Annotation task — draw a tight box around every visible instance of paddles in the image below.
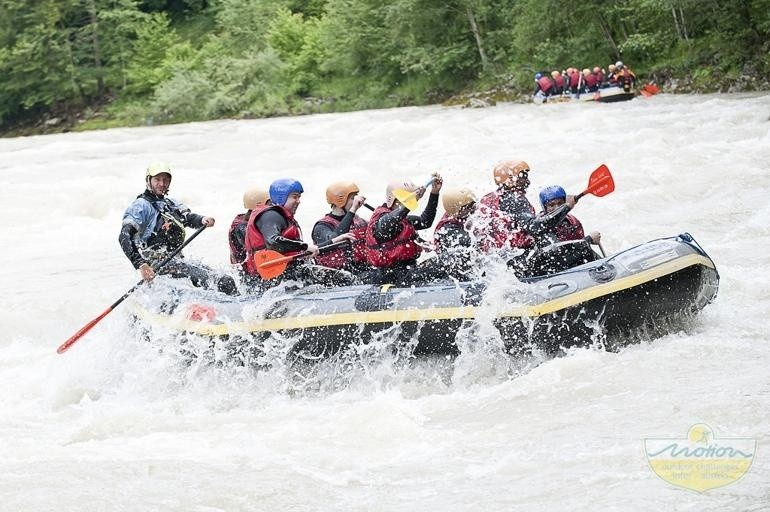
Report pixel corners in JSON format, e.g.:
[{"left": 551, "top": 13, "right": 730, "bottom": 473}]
[
  {"left": 255, "top": 236, "right": 366, "bottom": 279},
  {"left": 57, "top": 223, "right": 207, "bottom": 354},
  {"left": 634, "top": 78, "right": 660, "bottom": 97},
  {"left": 573, "top": 164, "right": 615, "bottom": 200},
  {"left": 392, "top": 177, "right": 437, "bottom": 211}
]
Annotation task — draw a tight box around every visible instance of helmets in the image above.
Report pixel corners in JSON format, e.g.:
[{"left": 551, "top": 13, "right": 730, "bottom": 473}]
[{"left": 536, "top": 61, "right": 624, "bottom": 80}]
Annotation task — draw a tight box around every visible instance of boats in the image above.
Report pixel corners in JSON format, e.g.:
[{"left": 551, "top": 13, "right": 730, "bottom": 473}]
[
  {"left": 132, "top": 236, "right": 722, "bottom": 376},
  {"left": 531, "top": 86, "right": 635, "bottom": 102}
]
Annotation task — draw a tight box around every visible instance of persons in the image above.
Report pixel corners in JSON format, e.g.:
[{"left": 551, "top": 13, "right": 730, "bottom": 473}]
[
  {"left": 531, "top": 61, "right": 635, "bottom": 97},
  {"left": 228, "top": 187, "right": 271, "bottom": 293},
  {"left": 537, "top": 186, "right": 601, "bottom": 244},
  {"left": 245, "top": 177, "right": 363, "bottom": 285},
  {"left": 119, "top": 162, "right": 241, "bottom": 297},
  {"left": 365, "top": 172, "right": 448, "bottom": 288},
  {"left": 433, "top": 185, "right": 479, "bottom": 282},
  {"left": 480, "top": 158, "right": 603, "bottom": 283},
  {"left": 311, "top": 180, "right": 433, "bottom": 285}
]
